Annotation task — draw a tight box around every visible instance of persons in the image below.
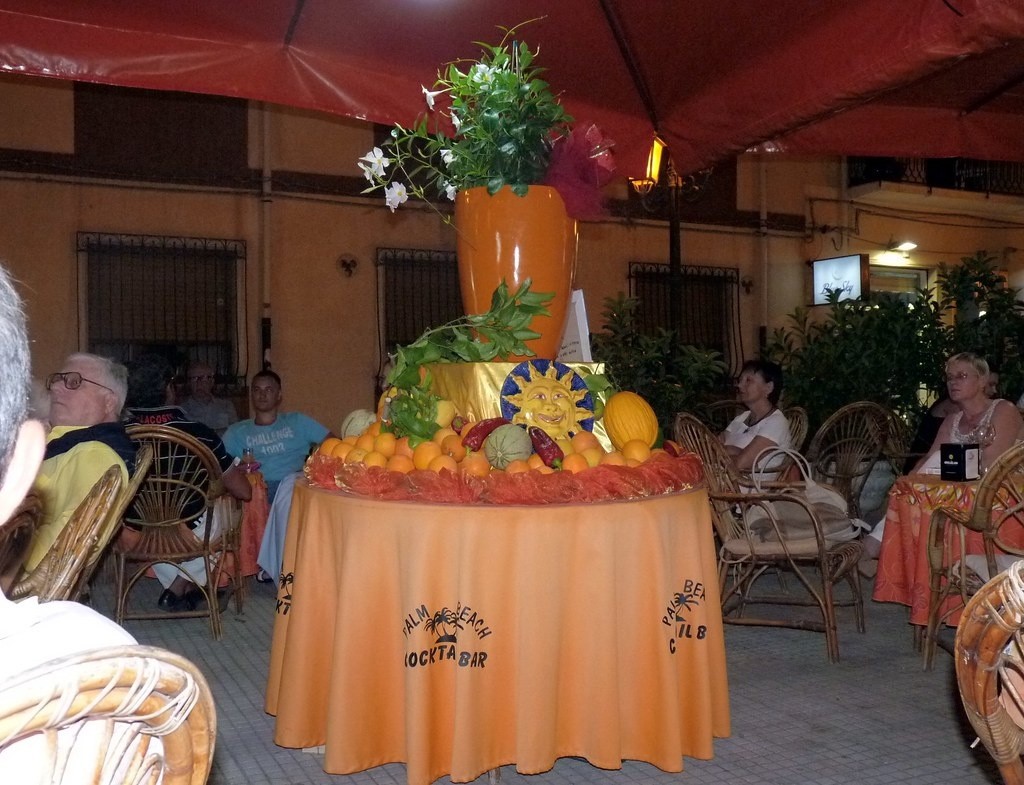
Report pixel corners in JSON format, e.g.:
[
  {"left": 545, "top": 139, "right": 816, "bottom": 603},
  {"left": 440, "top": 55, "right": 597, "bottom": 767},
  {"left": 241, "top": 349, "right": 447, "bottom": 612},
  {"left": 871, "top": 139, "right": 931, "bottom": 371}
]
[
  {"left": 116, "top": 357, "right": 252, "bottom": 613},
  {"left": 220, "top": 370, "right": 337, "bottom": 584},
  {"left": 1, "top": 352, "right": 137, "bottom": 600},
  {"left": 178, "top": 360, "right": 238, "bottom": 430},
  {"left": 0, "top": 264, "right": 163, "bottom": 785},
  {"left": 379, "top": 358, "right": 399, "bottom": 392},
  {"left": 838, "top": 350, "right": 1024, "bottom": 578},
  {"left": 713, "top": 357, "right": 792, "bottom": 518}
]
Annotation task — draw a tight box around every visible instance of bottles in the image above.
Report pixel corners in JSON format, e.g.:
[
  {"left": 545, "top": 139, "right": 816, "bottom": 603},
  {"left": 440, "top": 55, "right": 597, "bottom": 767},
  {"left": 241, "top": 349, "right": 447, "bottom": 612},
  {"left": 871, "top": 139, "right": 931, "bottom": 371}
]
[{"left": 242, "top": 449, "right": 255, "bottom": 476}]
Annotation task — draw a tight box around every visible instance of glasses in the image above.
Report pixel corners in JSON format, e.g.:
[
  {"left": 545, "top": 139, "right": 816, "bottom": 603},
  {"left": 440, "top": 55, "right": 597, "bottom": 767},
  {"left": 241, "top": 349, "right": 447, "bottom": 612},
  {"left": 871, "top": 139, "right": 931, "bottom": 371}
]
[
  {"left": 46, "top": 372, "right": 114, "bottom": 395},
  {"left": 942, "top": 371, "right": 980, "bottom": 383},
  {"left": 189, "top": 374, "right": 213, "bottom": 384}
]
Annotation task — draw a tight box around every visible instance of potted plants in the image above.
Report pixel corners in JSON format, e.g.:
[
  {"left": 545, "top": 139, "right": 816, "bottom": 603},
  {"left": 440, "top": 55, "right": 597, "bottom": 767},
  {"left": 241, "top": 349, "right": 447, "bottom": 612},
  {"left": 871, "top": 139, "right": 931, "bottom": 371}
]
[{"left": 752, "top": 286, "right": 958, "bottom": 519}]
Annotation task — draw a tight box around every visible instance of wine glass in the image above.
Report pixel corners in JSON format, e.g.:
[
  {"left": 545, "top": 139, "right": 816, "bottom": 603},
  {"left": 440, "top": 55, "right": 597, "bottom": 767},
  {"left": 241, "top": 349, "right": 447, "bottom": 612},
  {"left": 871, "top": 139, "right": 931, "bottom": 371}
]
[{"left": 968, "top": 425, "right": 997, "bottom": 477}]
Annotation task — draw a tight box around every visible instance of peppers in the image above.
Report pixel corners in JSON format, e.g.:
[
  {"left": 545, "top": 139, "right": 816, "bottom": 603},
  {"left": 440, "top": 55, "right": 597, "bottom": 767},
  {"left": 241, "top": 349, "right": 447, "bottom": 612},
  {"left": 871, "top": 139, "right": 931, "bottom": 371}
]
[
  {"left": 529, "top": 426, "right": 564, "bottom": 472},
  {"left": 461, "top": 417, "right": 512, "bottom": 454},
  {"left": 451, "top": 415, "right": 470, "bottom": 433}
]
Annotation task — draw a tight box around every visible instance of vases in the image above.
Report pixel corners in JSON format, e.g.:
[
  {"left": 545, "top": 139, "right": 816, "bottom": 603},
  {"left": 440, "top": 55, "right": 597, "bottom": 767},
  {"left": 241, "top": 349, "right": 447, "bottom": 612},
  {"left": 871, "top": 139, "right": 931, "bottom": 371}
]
[{"left": 452, "top": 184, "right": 573, "bottom": 362}]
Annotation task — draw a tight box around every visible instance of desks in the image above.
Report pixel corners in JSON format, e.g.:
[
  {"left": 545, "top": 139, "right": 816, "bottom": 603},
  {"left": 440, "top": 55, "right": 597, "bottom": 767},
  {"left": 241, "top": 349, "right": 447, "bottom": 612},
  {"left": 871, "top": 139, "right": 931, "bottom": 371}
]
[
  {"left": 872, "top": 474, "right": 1024, "bottom": 651},
  {"left": 263, "top": 476, "right": 732, "bottom": 785},
  {"left": 111, "top": 472, "right": 272, "bottom": 587}
]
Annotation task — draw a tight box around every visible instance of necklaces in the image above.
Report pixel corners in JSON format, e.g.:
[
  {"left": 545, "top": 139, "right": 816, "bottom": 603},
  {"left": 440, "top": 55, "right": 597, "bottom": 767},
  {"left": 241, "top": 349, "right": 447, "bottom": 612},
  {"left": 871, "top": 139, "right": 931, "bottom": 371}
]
[{"left": 743, "top": 407, "right": 773, "bottom": 433}]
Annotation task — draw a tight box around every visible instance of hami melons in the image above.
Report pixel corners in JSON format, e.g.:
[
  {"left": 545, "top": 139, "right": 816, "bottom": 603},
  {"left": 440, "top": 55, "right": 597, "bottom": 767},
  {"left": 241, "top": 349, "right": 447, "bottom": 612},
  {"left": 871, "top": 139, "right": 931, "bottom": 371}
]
[
  {"left": 341, "top": 408, "right": 376, "bottom": 439},
  {"left": 484, "top": 424, "right": 532, "bottom": 470}
]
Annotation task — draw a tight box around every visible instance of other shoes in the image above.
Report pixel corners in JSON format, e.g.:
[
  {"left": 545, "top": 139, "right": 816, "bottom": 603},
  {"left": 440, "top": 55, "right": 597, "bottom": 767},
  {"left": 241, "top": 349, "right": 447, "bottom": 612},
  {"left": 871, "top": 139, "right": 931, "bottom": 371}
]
[
  {"left": 176, "top": 587, "right": 203, "bottom": 611},
  {"left": 858, "top": 558, "right": 880, "bottom": 577},
  {"left": 257, "top": 570, "right": 275, "bottom": 583},
  {"left": 158, "top": 587, "right": 185, "bottom": 612}
]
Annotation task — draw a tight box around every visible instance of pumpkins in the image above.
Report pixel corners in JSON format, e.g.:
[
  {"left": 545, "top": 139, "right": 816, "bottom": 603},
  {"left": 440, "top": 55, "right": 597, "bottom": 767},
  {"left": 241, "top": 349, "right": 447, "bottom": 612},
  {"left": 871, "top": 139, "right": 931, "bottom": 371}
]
[{"left": 604, "top": 390, "right": 659, "bottom": 450}]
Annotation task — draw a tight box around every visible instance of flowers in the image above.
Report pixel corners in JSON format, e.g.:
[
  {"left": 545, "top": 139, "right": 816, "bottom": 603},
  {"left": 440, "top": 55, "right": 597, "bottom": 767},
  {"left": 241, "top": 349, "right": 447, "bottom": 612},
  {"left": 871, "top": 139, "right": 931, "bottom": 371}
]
[{"left": 356, "top": 14, "right": 574, "bottom": 249}]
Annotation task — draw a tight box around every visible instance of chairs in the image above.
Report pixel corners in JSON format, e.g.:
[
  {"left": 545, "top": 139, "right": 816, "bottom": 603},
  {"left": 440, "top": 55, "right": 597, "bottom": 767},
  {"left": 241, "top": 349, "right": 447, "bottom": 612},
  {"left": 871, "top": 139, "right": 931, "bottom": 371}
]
[
  {"left": 954, "top": 560, "right": 1024, "bottom": 785},
  {"left": 672, "top": 398, "right": 927, "bottom": 667},
  {"left": 924, "top": 441, "right": 1023, "bottom": 673},
  {"left": 0, "top": 443, "right": 154, "bottom": 604},
  {"left": 111, "top": 424, "right": 245, "bottom": 642},
  {"left": 0, "top": 644, "right": 217, "bottom": 785}
]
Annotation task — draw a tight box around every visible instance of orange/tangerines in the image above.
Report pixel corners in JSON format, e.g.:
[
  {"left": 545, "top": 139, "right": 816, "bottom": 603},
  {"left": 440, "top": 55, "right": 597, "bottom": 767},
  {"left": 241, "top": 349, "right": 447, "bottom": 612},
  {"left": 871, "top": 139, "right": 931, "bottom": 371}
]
[{"left": 317, "top": 419, "right": 666, "bottom": 477}]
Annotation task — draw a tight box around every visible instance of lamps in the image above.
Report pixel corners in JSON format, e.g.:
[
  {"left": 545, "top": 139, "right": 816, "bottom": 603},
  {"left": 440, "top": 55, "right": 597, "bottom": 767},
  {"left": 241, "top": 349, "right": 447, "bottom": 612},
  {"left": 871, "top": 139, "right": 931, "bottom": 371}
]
[{"left": 889, "top": 241, "right": 917, "bottom": 252}]
